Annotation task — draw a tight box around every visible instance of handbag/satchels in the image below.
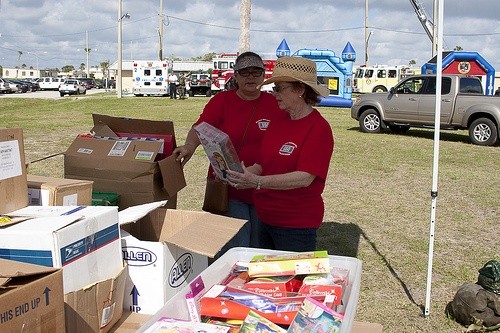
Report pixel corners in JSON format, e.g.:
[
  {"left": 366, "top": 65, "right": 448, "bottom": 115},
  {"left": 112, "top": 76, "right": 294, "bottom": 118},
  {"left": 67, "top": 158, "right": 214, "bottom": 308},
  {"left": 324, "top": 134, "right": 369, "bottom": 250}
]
[
  {"left": 478, "top": 260, "right": 500, "bottom": 294},
  {"left": 202, "top": 178, "right": 229, "bottom": 214}
]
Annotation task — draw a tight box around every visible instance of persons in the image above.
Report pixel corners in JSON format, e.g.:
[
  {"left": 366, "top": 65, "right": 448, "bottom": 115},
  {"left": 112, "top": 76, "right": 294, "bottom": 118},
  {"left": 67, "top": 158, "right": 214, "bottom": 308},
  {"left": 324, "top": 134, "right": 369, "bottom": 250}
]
[
  {"left": 225, "top": 56, "right": 335, "bottom": 252},
  {"left": 171, "top": 51, "right": 280, "bottom": 257},
  {"left": 168, "top": 70, "right": 186, "bottom": 100}
]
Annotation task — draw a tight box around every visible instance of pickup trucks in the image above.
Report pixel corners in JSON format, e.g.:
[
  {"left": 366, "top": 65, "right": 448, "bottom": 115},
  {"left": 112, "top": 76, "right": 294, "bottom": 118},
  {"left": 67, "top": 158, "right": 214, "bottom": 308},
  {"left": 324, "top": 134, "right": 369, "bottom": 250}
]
[
  {"left": 58, "top": 79, "right": 86, "bottom": 97},
  {"left": 351, "top": 74, "right": 500, "bottom": 146}
]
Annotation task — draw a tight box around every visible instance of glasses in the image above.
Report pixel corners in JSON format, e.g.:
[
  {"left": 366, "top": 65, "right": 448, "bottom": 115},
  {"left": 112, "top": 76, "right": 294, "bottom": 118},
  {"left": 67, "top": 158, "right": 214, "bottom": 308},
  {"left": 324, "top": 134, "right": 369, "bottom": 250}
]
[
  {"left": 272, "top": 83, "right": 298, "bottom": 92},
  {"left": 236, "top": 70, "right": 263, "bottom": 77}
]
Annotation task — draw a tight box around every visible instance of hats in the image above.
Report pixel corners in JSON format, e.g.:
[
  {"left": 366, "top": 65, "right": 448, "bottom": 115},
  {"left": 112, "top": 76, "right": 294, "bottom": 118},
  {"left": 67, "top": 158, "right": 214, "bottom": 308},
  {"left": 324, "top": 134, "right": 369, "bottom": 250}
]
[
  {"left": 233, "top": 55, "right": 265, "bottom": 71},
  {"left": 256, "top": 56, "right": 330, "bottom": 99}
]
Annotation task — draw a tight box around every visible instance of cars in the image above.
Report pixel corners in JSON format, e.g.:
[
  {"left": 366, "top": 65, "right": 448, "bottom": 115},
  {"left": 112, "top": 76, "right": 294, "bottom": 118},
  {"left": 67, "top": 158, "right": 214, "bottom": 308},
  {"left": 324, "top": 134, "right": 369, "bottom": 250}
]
[
  {"left": 67, "top": 77, "right": 116, "bottom": 90},
  {"left": 0, "top": 78, "right": 38, "bottom": 94},
  {"left": 175, "top": 72, "right": 211, "bottom": 97}
]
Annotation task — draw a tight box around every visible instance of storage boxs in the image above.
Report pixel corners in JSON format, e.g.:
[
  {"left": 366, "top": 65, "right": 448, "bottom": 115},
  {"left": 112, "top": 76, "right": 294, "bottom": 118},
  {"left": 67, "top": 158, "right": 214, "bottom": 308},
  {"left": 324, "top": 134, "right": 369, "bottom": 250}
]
[{"left": 0, "top": 113, "right": 361, "bottom": 333}]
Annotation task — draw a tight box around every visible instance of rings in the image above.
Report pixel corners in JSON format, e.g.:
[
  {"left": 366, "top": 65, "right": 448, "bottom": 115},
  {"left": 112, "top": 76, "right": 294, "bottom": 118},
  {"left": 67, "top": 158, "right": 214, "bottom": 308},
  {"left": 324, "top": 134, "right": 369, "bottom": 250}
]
[{"left": 234, "top": 183, "right": 238, "bottom": 188}]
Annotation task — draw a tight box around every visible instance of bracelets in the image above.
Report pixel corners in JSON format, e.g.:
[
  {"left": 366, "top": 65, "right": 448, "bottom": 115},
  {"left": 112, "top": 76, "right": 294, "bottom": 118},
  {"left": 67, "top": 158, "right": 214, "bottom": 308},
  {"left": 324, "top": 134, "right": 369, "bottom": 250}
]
[{"left": 255, "top": 176, "right": 261, "bottom": 191}]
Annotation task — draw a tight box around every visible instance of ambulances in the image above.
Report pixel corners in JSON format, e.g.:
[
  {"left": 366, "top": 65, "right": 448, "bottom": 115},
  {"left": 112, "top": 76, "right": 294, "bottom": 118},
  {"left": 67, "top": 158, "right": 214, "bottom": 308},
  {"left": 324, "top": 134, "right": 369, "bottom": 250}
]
[
  {"left": 352, "top": 64, "right": 421, "bottom": 93},
  {"left": 34, "top": 77, "right": 67, "bottom": 91}
]
